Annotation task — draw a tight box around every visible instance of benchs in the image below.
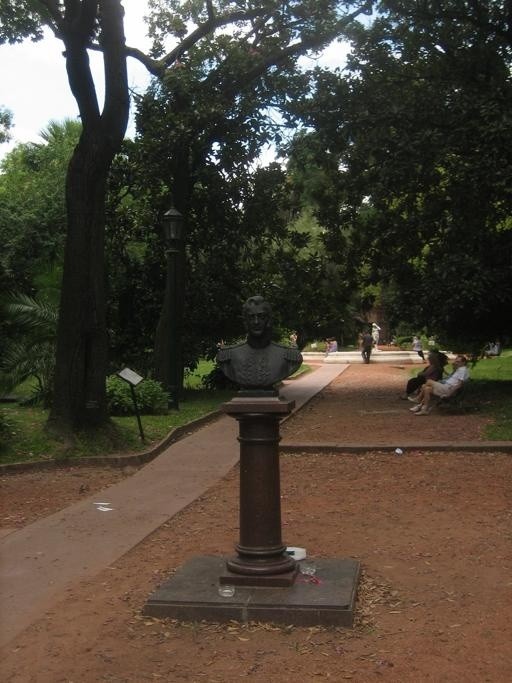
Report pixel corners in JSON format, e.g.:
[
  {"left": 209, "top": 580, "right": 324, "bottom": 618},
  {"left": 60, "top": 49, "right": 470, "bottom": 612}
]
[{"left": 438, "top": 382, "right": 484, "bottom": 415}]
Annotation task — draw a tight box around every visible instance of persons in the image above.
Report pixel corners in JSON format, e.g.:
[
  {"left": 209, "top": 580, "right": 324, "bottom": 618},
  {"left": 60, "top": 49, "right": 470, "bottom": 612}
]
[
  {"left": 320, "top": 335, "right": 337, "bottom": 352},
  {"left": 357, "top": 329, "right": 372, "bottom": 363},
  {"left": 372, "top": 323, "right": 381, "bottom": 351},
  {"left": 400, "top": 350, "right": 470, "bottom": 415},
  {"left": 412, "top": 336, "right": 424, "bottom": 359},
  {"left": 216, "top": 296, "right": 304, "bottom": 389},
  {"left": 480, "top": 338, "right": 501, "bottom": 359},
  {"left": 387, "top": 335, "right": 392, "bottom": 347},
  {"left": 290, "top": 331, "right": 297, "bottom": 343}
]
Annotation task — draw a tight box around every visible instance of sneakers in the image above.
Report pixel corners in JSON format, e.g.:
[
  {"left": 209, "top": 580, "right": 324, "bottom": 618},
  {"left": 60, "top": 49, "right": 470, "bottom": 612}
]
[{"left": 408, "top": 396, "right": 429, "bottom": 415}]
